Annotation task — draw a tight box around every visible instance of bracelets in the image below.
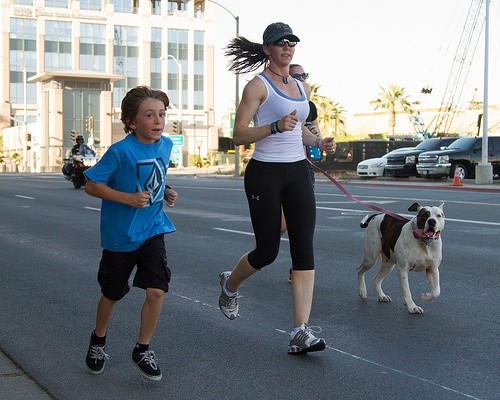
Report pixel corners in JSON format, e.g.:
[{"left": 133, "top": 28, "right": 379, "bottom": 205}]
[
  {"left": 165, "top": 184, "right": 171, "bottom": 189},
  {"left": 271, "top": 119, "right": 283, "bottom": 134},
  {"left": 315, "top": 137, "right": 321, "bottom": 148}
]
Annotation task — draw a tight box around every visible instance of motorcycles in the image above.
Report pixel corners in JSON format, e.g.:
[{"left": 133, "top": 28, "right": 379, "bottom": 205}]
[
  {"left": 61, "top": 152, "right": 73, "bottom": 181},
  {"left": 68, "top": 143, "right": 99, "bottom": 189}
]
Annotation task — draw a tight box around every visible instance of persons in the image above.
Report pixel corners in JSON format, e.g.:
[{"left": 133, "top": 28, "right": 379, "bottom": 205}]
[
  {"left": 71, "top": 135, "right": 96, "bottom": 182},
  {"left": 83, "top": 85, "right": 178, "bottom": 382},
  {"left": 218, "top": 22, "right": 337, "bottom": 354},
  {"left": 259, "top": 64, "right": 323, "bottom": 281}
]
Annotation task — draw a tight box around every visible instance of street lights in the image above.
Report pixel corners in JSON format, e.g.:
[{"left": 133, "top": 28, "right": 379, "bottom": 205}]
[
  {"left": 159, "top": 54, "right": 184, "bottom": 168},
  {"left": 8, "top": 61, "right": 29, "bottom": 173}
]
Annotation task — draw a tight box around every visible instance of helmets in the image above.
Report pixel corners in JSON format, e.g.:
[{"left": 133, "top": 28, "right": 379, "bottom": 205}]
[{"left": 76, "top": 135, "right": 84, "bottom": 143}]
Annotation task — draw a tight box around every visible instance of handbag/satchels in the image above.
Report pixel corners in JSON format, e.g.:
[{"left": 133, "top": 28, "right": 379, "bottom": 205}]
[{"left": 306, "top": 145, "right": 321, "bottom": 160}]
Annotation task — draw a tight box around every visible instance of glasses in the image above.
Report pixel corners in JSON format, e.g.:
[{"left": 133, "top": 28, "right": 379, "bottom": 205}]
[
  {"left": 291, "top": 73, "right": 309, "bottom": 80},
  {"left": 267, "top": 36, "right": 297, "bottom": 47}
]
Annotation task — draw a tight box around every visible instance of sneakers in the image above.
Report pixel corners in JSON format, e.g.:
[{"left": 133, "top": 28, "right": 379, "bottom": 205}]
[
  {"left": 86, "top": 333, "right": 107, "bottom": 374},
  {"left": 132, "top": 347, "right": 162, "bottom": 381},
  {"left": 219, "top": 271, "right": 239, "bottom": 320},
  {"left": 287, "top": 324, "right": 326, "bottom": 355}
]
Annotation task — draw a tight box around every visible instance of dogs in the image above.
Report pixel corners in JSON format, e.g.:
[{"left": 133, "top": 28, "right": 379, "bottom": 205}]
[{"left": 355, "top": 199, "right": 446, "bottom": 314}]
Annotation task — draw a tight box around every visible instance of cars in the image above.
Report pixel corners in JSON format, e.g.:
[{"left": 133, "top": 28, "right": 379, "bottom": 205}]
[{"left": 357, "top": 147, "right": 414, "bottom": 177}]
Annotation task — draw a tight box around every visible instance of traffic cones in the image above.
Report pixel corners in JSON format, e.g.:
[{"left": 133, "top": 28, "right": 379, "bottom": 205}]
[{"left": 450, "top": 166, "right": 464, "bottom": 186}]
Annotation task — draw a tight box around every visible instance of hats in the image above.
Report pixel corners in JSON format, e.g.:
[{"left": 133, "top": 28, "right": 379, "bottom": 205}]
[{"left": 263, "top": 22, "right": 300, "bottom": 45}]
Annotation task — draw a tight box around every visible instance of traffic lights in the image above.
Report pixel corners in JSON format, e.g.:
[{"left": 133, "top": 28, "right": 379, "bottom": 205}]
[
  {"left": 85, "top": 116, "right": 93, "bottom": 132},
  {"left": 70, "top": 130, "right": 76, "bottom": 143},
  {"left": 27, "top": 134, "right": 31, "bottom": 141},
  {"left": 173, "top": 120, "right": 178, "bottom": 133}
]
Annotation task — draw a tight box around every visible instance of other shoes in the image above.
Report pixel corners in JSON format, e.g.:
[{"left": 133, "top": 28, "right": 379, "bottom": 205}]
[{"left": 288, "top": 268, "right": 292, "bottom": 282}]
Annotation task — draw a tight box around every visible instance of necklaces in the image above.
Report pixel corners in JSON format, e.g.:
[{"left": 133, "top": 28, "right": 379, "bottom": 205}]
[{"left": 267, "top": 67, "right": 289, "bottom": 84}]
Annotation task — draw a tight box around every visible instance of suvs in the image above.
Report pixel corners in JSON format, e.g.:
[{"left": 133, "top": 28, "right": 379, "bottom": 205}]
[
  {"left": 384, "top": 137, "right": 461, "bottom": 179},
  {"left": 415, "top": 136, "right": 500, "bottom": 179}
]
